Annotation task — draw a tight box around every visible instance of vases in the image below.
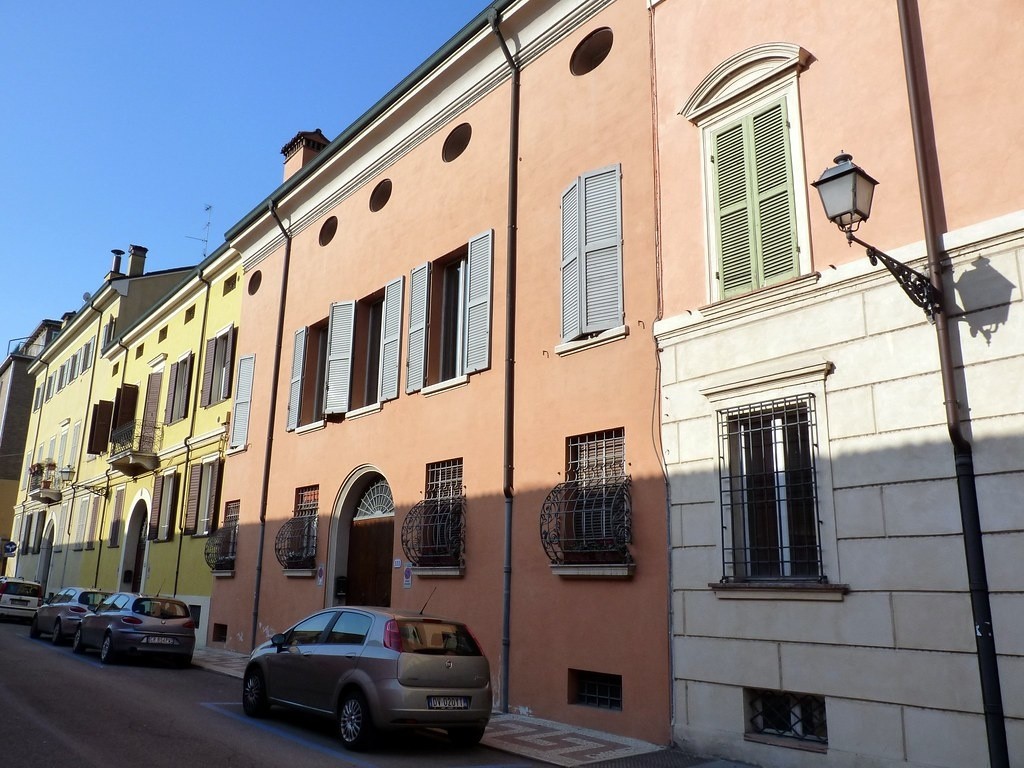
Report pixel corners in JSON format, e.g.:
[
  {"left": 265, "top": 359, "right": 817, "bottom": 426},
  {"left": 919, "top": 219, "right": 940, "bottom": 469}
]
[{"left": 43, "top": 481, "right": 51, "bottom": 489}]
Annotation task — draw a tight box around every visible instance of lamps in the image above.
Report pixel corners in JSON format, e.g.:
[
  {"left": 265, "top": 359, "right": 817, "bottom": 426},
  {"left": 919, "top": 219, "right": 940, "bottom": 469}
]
[
  {"left": 61, "top": 465, "right": 108, "bottom": 497},
  {"left": 809, "top": 150, "right": 944, "bottom": 324}
]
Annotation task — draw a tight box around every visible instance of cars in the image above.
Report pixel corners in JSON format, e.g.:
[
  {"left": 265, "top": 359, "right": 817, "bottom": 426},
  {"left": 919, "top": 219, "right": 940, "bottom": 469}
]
[
  {"left": 30, "top": 586, "right": 115, "bottom": 646},
  {"left": 242, "top": 605, "right": 493, "bottom": 753},
  {"left": 71, "top": 592, "right": 196, "bottom": 669},
  {"left": 0, "top": 576, "right": 44, "bottom": 625}
]
